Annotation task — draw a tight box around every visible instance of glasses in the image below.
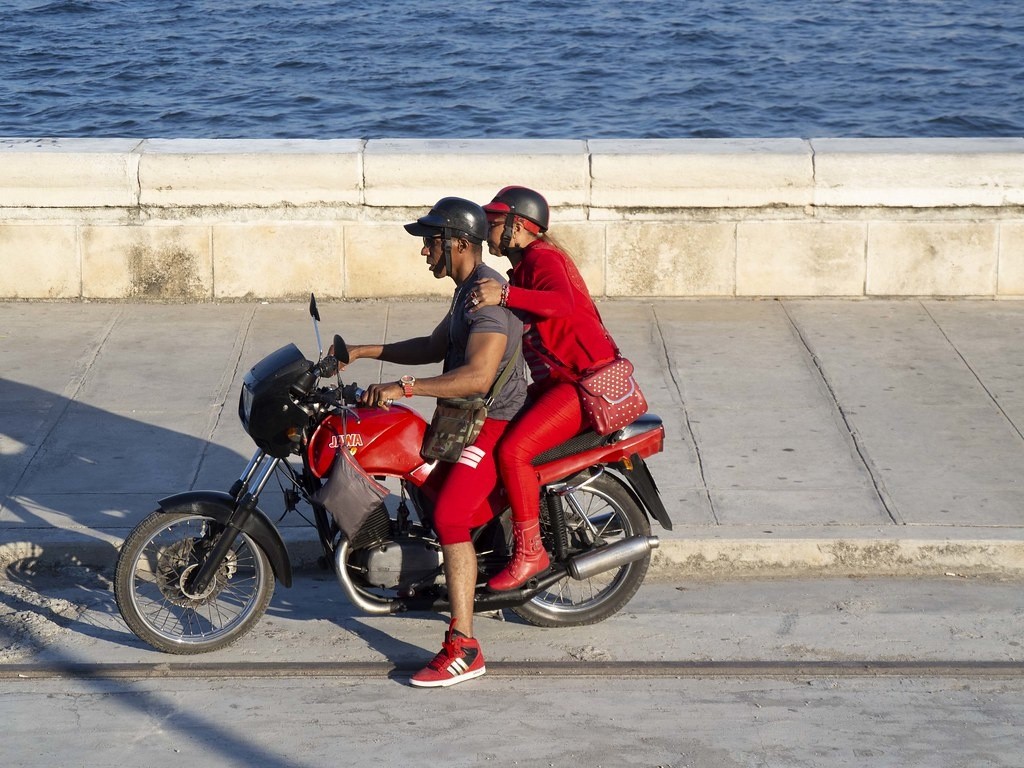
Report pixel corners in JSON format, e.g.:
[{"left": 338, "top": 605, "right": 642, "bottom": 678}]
[
  {"left": 488, "top": 220, "right": 524, "bottom": 233},
  {"left": 423, "top": 235, "right": 458, "bottom": 248}
]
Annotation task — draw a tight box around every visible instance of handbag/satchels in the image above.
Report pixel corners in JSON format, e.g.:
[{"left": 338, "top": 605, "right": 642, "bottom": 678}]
[
  {"left": 577, "top": 358, "right": 648, "bottom": 437},
  {"left": 306, "top": 444, "right": 392, "bottom": 540},
  {"left": 421, "top": 398, "right": 487, "bottom": 463}
]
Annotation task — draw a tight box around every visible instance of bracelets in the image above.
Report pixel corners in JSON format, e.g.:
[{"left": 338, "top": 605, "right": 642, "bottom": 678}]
[{"left": 500, "top": 284, "right": 509, "bottom": 307}]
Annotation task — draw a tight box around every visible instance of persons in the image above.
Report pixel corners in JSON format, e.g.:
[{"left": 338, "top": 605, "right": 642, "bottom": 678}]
[
  {"left": 327, "top": 197, "right": 527, "bottom": 689},
  {"left": 464, "top": 185, "right": 619, "bottom": 593}
]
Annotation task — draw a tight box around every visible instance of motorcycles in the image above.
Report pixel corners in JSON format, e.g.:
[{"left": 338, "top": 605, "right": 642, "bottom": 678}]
[{"left": 112, "top": 293, "right": 675, "bottom": 656}]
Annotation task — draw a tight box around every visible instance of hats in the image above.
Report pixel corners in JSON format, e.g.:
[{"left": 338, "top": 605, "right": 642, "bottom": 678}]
[
  {"left": 484, "top": 211, "right": 540, "bottom": 235},
  {"left": 404, "top": 221, "right": 481, "bottom": 244}
]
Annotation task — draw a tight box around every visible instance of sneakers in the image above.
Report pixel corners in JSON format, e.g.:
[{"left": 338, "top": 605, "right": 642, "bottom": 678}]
[{"left": 408, "top": 618, "right": 486, "bottom": 687}]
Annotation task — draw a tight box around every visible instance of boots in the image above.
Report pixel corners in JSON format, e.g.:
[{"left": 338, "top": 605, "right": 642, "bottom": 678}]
[{"left": 487, "top": 517, "right": 551, "bottom": 593}]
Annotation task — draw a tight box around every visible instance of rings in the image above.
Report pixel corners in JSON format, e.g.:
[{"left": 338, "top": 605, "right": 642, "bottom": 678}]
[
  {"left": 377, "top": 401, "right": 386, "bottom": 407},
  {"left": 472, "top": 298, "right": 479, "bottom": 305},
  {"left": 470, "top": 291, "right": 477, "bottom": 299}
]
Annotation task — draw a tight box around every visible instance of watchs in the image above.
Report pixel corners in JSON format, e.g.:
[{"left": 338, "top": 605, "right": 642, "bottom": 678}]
[{"left": 399, "top": 375, "right": 416, "bottom": 397}]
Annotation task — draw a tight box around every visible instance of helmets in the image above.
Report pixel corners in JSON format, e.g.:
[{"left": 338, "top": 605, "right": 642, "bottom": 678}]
[
  {"left": 482, "top": 186, "right": 549, "bottom": 230},
  {"left": 418, "top": 196, "right": 487, "bottom": 241}
]
